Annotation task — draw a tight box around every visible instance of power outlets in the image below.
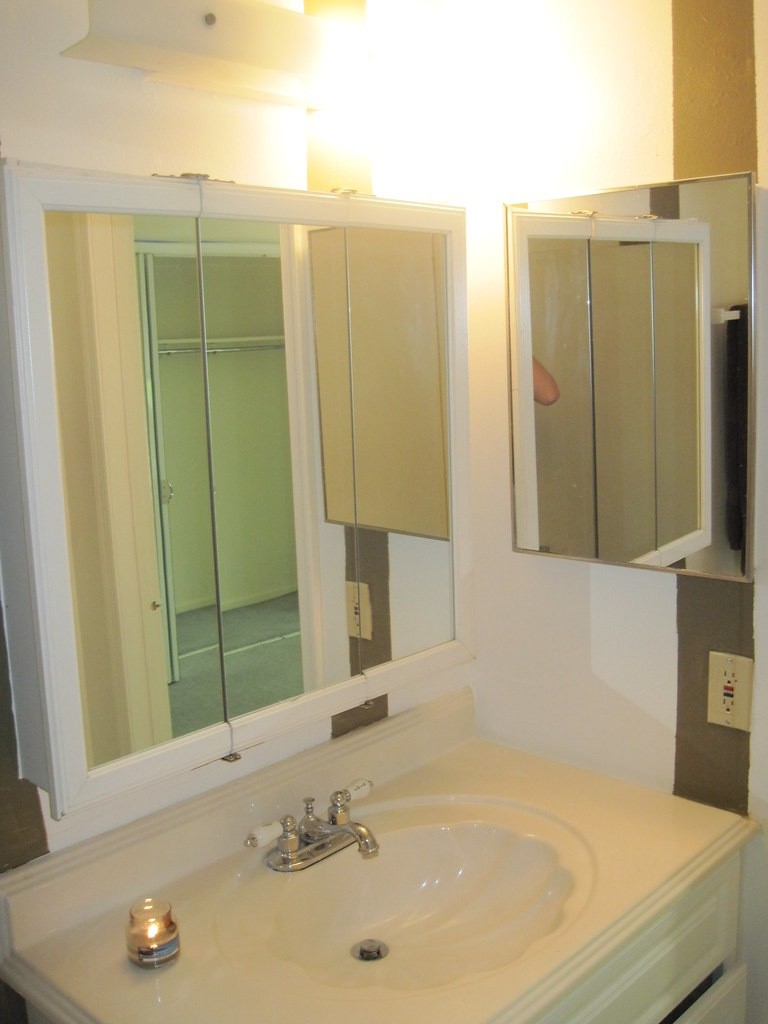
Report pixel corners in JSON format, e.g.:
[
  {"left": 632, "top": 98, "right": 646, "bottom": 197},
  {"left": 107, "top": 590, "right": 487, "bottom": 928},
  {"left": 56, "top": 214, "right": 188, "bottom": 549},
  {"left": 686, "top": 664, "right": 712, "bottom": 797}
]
[
  {"left": 706, "top": 650, "right": 755, "bottom": 734},
  {"left": 344, "top": 582, "right": 377, "bottom": 644}
]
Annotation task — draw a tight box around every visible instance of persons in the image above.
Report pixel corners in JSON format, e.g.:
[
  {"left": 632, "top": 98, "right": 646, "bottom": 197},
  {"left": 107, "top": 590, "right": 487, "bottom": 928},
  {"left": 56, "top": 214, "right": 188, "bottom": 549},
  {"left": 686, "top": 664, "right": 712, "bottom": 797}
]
[{"left": 532, "top": 355, "right": 560, "bottom": 406}]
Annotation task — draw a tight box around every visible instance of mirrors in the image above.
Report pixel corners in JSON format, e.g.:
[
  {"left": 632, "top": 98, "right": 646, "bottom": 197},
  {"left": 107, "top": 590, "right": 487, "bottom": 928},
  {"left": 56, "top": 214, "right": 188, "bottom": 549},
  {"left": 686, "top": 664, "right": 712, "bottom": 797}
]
[
  {"left": 309, "top": 228, "right": 455, "bottom": 542},
  {"left": 42, "top": 206, "right": 457, "bottom": 773},
  {"left": 505, "top": 170, "right": 756, "bottom": 587}
]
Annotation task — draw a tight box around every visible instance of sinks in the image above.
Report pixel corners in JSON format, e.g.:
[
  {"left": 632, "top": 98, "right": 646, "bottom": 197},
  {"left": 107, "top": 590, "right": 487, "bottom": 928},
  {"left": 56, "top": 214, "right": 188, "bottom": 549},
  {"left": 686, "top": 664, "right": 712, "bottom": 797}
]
[{"left": 209, "top": 784, "right": 600, "bottom": 1000}]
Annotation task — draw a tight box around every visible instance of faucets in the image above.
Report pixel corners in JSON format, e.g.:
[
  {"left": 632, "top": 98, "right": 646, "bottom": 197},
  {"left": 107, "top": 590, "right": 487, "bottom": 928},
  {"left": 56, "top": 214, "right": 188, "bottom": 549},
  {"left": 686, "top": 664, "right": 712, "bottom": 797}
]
[{"left": 298, "top": 795, "right": 380, "bottom": 859}]
